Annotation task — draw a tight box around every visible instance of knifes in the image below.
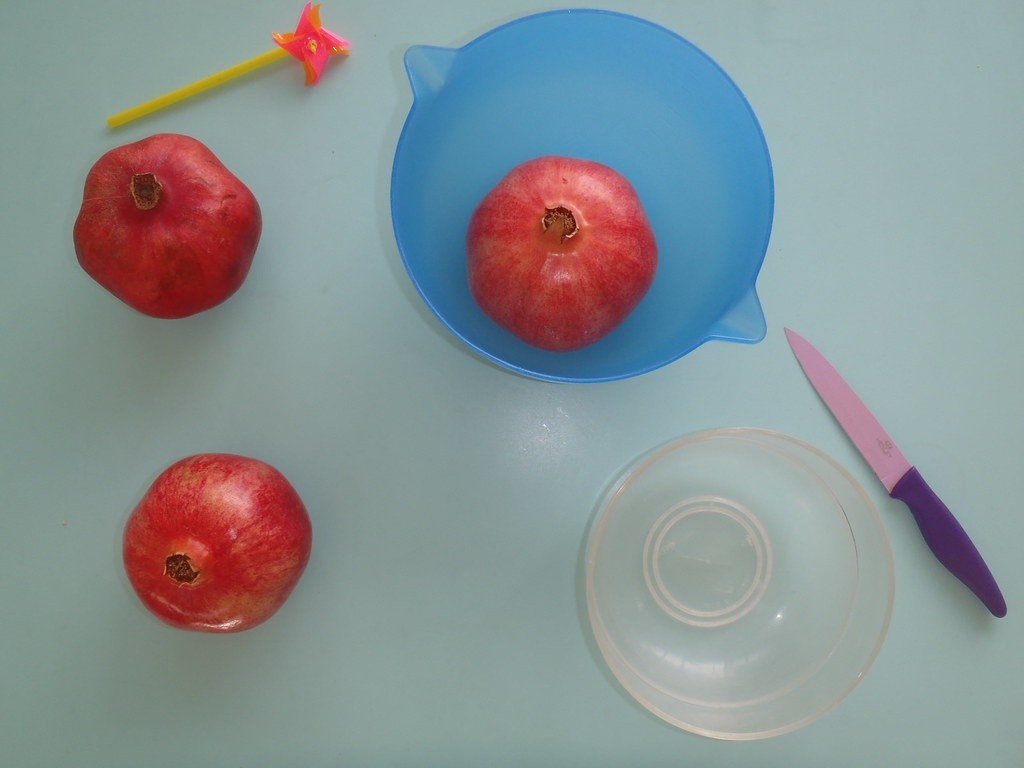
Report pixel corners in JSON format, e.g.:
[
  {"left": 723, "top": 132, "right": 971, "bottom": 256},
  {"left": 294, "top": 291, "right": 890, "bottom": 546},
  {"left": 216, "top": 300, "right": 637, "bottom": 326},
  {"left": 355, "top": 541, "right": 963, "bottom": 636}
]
[{"left": 782, "top": 328, "right": 1008, "bottom": 619}]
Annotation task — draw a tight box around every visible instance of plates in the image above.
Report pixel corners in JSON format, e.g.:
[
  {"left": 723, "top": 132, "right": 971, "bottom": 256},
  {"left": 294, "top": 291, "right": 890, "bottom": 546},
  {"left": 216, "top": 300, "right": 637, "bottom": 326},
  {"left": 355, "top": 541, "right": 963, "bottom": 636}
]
[
  {"left": 586, "top": 429, "right": 893, "bottom": 740},
  {"left": 392, "top": 9, "right": 775, "bottom": 382}
]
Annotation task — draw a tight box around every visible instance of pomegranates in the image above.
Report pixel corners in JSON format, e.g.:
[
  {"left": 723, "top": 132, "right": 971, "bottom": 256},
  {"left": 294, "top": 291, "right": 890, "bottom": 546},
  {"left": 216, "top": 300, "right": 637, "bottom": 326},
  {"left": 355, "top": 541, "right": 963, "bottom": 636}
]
[
  {"left": 467, "top": 157, "right": 659, "bottom": 350},
  {"left": 73, "top": 136, "right": 262, "bottom": 316},
  {"left": 123, "top": 453, "right": 312, "bottom": 635}
]
